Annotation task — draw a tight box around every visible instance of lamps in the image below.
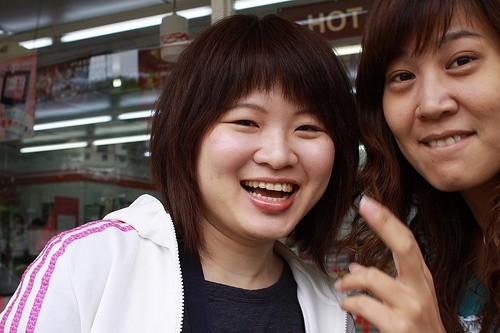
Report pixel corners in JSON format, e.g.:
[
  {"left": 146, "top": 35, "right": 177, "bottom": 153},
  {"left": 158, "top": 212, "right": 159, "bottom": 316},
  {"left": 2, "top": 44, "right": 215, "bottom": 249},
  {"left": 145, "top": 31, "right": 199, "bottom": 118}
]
[{"left": 160, "top": 0, "right": 189, "bottom": 63}]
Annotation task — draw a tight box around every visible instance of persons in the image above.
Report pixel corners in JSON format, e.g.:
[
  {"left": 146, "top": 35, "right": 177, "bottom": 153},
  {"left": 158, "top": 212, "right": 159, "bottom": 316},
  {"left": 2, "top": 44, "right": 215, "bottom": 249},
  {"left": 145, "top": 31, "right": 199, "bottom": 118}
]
[
  {"left": 0, "top": 214, "right": 51, "bottom": 274},
  {"left": 0, "top": 14, "right": 446, "bottom": 333},
  {"left": 335, "top": 0, "right": 500, "bottom": 333}
]
